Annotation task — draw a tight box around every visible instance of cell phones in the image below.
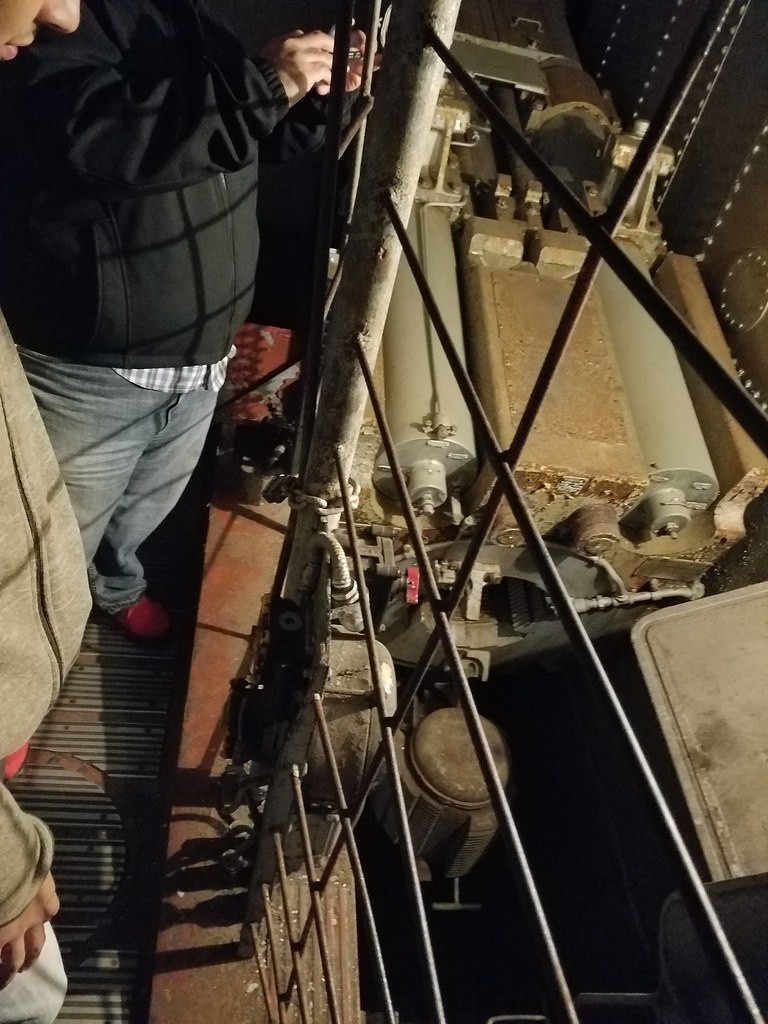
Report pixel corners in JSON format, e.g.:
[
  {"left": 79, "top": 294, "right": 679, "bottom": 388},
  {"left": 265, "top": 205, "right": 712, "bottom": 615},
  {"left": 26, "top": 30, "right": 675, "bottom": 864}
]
[{"left": 348, "top": 48, "right": 362, "bottom": 59}]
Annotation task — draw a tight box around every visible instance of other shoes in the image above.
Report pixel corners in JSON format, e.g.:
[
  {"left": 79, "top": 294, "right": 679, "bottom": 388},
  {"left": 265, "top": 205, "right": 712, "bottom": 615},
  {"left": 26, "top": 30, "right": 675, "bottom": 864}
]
[
  {"left": 2, "top": 743, "right": 34, "bottom": 784},
  {"left": 100, "top": 593, "right": 173, "bottom": 648}
]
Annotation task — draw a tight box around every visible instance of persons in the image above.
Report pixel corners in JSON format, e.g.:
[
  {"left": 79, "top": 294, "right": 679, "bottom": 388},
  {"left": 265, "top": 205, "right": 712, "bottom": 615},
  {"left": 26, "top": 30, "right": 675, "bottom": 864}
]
[{"left": 0, "top": 0, "right": 382, "bottom": 1024}]
